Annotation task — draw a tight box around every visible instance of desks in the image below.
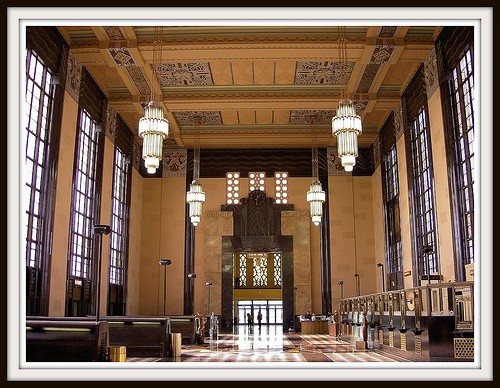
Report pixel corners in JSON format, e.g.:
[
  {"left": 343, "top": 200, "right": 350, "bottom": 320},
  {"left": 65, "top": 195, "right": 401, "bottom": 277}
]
[{"left": 301, "top": 321, "right": 328, "bottom": 335}]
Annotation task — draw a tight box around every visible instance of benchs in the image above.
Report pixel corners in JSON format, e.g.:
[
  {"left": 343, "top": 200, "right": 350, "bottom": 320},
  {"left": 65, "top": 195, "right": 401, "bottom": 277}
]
[{"left": 26, "top": 314, "right": 222, "bottom": 362}]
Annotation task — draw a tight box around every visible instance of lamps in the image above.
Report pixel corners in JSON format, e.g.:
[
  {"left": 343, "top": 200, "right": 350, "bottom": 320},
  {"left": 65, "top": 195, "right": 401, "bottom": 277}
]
[
  {"left": 137, "top": 27, "right": 169, "bottom": 176},
  {"left": 331, "top": 26, "right": 362, "bottom": 173},
  {"left": 306, "top": 115, "right": 326, "bottom": 227},
  {"left": 186, "top": 116, "right": 206, "bottom": 226}
]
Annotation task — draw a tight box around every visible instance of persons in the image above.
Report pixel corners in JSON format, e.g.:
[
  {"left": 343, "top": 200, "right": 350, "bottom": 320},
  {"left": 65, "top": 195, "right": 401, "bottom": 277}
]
[
  {"left": 305, "top": 311, "right": 312, "bottom": 320},
  {"left": 248, "top": 314, "right": 253, "bottom": 328},
  {"left": 257, "top": 312, "right": 262, "bottom": 326}
]
